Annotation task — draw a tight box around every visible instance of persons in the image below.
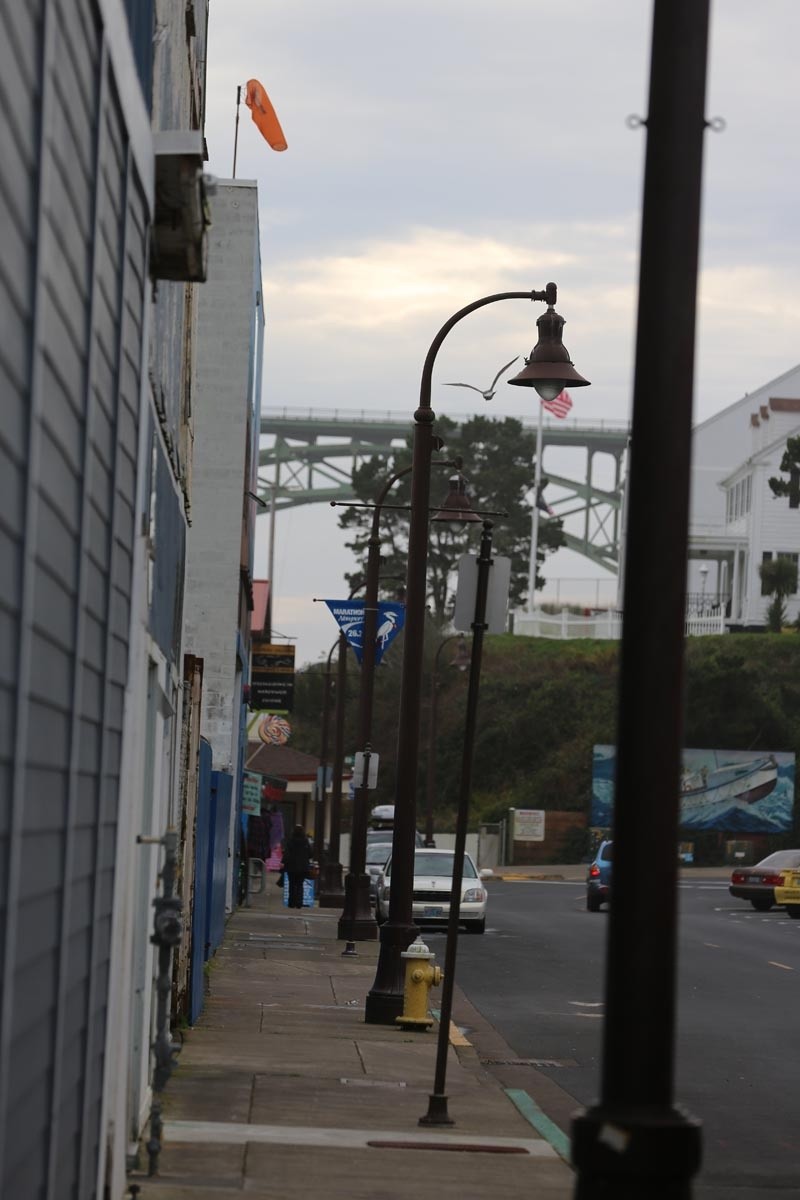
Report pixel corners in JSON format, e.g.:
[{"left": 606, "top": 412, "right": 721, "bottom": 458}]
[{"left": 279, "top": 827, "right": 312, "bottom": 909}]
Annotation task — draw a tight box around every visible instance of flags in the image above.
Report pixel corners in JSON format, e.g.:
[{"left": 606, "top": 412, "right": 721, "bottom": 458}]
[{"left": 542, "top": 389, "right": 573, "bottom": 420}]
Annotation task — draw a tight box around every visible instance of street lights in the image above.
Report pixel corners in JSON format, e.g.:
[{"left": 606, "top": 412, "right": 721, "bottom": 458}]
[
  {"left": 364, "top": 282, "right": 592, "bottom": 1025},
  {"left": 337, "top": 454, "right": 487, "bottom": 941},
  {"left": 318, "top": 574, "right": 432, "bottom": 909},
  {"left": 312, "top": 634, "right": 390, "bottom": 901}
]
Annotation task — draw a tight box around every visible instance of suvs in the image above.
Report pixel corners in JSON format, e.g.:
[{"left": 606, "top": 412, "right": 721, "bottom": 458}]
[{"left": 365, "top": 805, "right": 436, "bottom": 848}]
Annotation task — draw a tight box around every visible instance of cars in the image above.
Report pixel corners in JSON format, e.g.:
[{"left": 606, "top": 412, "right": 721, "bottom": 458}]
[
  {"left": 729, "top": 848, "right": 800, "bottom": 911},
  {"left": 364, "top": 842, "right": 396, "bottom": 907},
  {"left": 773, "top": 866, "right": 800, "bottom": 919},
  {"left": 369, "top": 847, "right": 494, "bottom": 934},
  {"left": 587, "top": 837, "right": 614, "bottom": 913}
]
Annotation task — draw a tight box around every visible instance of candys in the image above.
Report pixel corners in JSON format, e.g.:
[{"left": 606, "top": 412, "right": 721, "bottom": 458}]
[{"left": 245, "top": 714, "right": 291, "bottom": 769}]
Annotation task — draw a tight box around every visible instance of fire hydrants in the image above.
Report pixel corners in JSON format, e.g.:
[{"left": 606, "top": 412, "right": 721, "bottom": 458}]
[{"left": 393, "top": 936, "right": 445, "bottom": 1033}]
